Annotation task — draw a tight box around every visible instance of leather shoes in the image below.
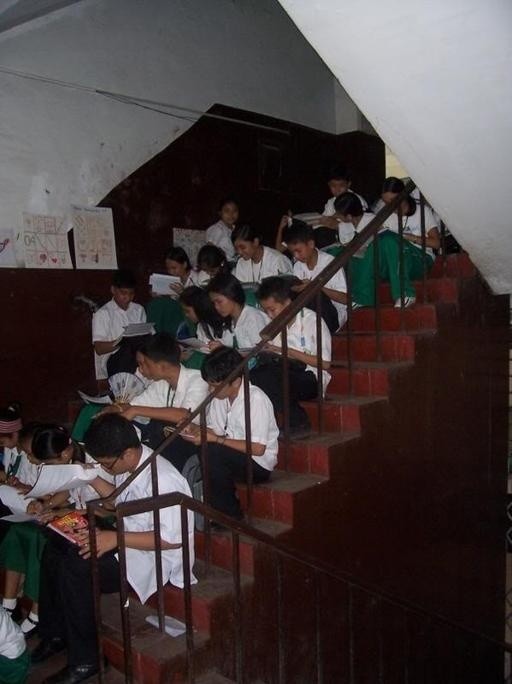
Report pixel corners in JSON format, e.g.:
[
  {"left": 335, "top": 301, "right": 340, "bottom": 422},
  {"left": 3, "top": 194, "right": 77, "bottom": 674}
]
[
  {"left": 30, "top": 640, "right": 70, "bottom": 670},
  {"left": 19, "top": 617, "right": 39, "bottom": 641},
  {"left": 2, "top": 600, "right": 24, "bottom": 623},
  {"left": 207, "top": 520, "right": 229, "bottom": 534},
  {"left": 278, "top": 422, "right": 313, "bottom": 443},
  {"left": 40, "top": 653, "right": 111, "bottom": 684}
]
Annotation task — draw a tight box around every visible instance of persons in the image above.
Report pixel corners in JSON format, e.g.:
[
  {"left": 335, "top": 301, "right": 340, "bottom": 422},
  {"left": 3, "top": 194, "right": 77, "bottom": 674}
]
[
  {"left": 2, "top": 333, "right": 280, "bottom": 684},
  {"left": 91, "top": 174, "right": 441, "bottom": 439}
]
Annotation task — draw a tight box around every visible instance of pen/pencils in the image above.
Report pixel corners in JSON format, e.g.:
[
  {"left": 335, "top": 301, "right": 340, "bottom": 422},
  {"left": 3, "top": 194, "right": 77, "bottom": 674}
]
[{"left": 185, "top": 408, "right": 191, "bottom": 434}]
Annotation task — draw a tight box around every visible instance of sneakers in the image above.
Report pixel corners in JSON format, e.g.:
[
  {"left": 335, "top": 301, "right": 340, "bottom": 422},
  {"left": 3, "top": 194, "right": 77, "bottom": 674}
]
[
  {"left": 394, "top": 297, "right": 418, "bottom": 308},
  {"left": 350, "top": 300, "right": 365, "bottom": 311}
]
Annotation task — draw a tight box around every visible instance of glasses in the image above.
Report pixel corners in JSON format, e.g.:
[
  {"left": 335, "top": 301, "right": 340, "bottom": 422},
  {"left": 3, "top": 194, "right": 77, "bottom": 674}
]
[{"left": 94, "top": 450, "right": 125, "bottom": 472}]
